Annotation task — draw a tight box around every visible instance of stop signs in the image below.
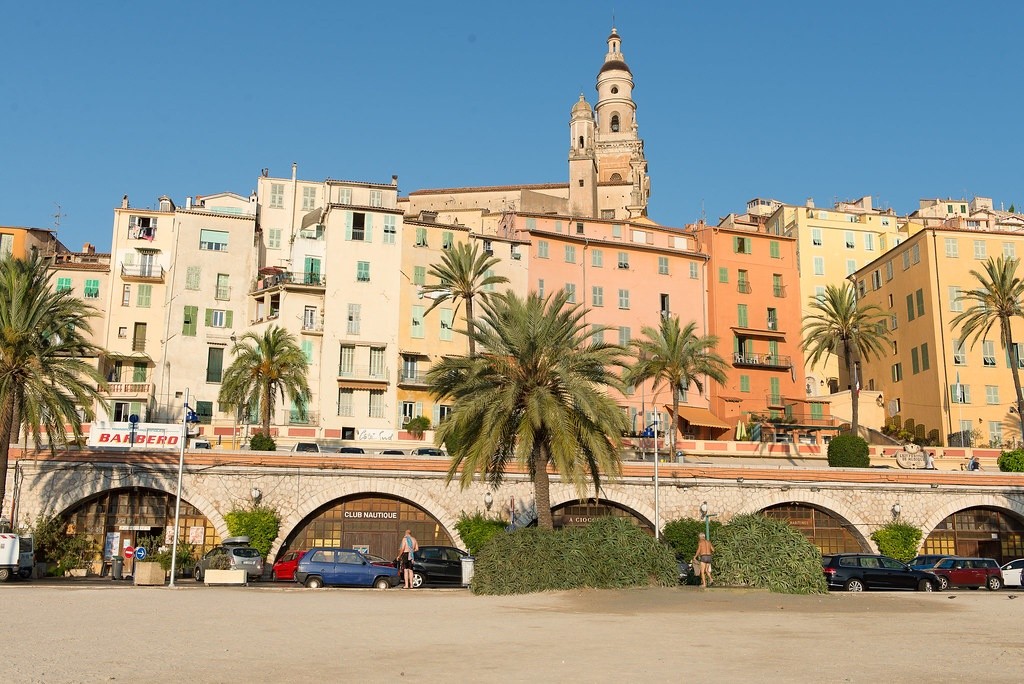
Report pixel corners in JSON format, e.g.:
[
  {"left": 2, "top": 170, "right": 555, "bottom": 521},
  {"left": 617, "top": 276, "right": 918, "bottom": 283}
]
[{"left": 125, "top": 547, "right": 134, "bottom": 558}]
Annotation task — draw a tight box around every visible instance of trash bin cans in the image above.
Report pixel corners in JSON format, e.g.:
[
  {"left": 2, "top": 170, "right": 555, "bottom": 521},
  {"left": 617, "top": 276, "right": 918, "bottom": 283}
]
[{"left": 460, "top": 557, "right": 475, "bottom": 588}]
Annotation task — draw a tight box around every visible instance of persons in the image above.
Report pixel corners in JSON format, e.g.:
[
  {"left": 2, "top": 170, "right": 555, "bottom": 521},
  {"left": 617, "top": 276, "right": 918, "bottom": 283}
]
[
  {"left": 396, "top": 529, "right": 419, "bottom": 589},
  {"left": 972, "top": 457, "right": 986, "bottom": 472},
  {"left": 926, "top": 452, "right": 935, "bottom": 470},
  {"left": 693, "top": 532, "right": 715, "bottom": 589},
  {"left": 123, "top": 412, "right": 129, "bottom": 421},
  {"left": 880, "top": 450, "right": 886, "bottom": 457},
  {"left": 967, "top": 455, "right": 976, "bottom": 471},
  {"left": 1011, "top": 436, "right": 1016, "bottom": 448},
  {"left": 944, "top": 452, "right": 946, "bottom": 457}
]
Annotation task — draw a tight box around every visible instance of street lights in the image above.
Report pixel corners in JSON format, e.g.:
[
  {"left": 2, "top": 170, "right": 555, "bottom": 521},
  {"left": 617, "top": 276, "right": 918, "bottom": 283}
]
[
  {"left": 642, "top": 406, "right": 660, "bottom": 541},
  {"left": 167, "top": 387, "right": 201, "bottom": 588}
]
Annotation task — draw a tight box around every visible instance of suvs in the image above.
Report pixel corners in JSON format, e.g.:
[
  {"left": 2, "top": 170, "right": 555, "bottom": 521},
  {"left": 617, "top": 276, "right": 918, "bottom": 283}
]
[
  {"left": 194, "top": 536, "right": 264, "bottom": 581},
  {"left": 394, "top": 544, "right": 471, "bottom": 588}
]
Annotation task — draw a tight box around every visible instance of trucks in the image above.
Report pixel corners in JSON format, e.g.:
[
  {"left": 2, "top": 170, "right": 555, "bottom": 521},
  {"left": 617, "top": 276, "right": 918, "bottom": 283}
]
[
  {"left": 0, "top": 533, "right": 34, "bottom": 582},
  {"left": 88, "top": 420, "right": 213, "bottom": 452}
]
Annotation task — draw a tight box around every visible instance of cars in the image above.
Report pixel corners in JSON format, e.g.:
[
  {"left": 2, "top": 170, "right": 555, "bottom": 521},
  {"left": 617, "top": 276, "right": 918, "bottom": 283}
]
[
  {"left": 1000, "top": 558, "right": 1024, "bottom": 589},
  {"left": 818, "top": 553, "right": 942, "bottom": 595},
  {"left": 297, "top": 546, "right": 400, "bottom": 590},
  {"left": 674, "top": 563, "right": 701, "bottom": 586},
  {"left": 331, "top": 554, "right": 394, "bottom": 568},
  {"left": 901, "top": 554, "right": 980, "bottom": 589},
  {"left": 922, "top": 557, "right": 1004, "bottom": 591},
  {"left": 272, "top": 550, "right": 326, "bottom": 582}
]
